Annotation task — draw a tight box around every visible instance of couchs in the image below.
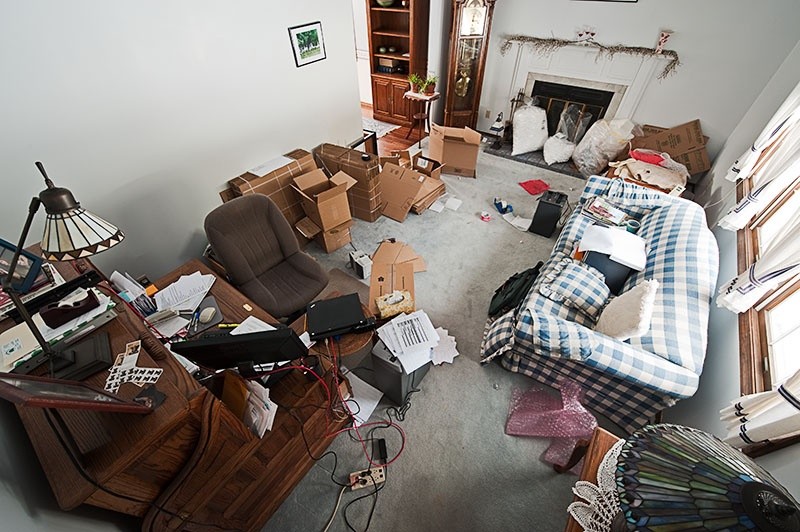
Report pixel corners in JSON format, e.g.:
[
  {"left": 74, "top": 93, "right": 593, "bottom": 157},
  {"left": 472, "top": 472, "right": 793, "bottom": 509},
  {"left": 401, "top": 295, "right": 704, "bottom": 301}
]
[{"left": 500, "top": 174, "right": 718, "bottom": 435}]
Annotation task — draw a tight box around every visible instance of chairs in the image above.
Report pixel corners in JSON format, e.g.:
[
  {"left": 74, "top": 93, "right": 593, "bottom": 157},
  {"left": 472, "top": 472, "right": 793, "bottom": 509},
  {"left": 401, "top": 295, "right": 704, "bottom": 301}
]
[{"left": 205, "top": 193, "right": 330, "bottom": 327}]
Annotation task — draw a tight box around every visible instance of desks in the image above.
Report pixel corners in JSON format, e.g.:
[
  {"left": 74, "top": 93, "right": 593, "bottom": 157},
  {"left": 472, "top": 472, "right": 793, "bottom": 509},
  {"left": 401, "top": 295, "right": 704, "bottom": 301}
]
[
  {"left": 0, "top": 240, "right": 353, "bottom": 532},
  {"left": 303, "top": 301, "right": 376, "bottom": 357},
  {"left": 402, "top": 90, "right": 441, "bottom": 149}
]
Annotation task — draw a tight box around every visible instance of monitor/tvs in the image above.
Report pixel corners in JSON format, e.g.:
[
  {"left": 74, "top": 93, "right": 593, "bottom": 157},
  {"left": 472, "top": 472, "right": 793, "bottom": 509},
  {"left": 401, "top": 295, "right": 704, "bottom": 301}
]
[{"left": 170, "top": 328, "right": 309, "bottom": 387}]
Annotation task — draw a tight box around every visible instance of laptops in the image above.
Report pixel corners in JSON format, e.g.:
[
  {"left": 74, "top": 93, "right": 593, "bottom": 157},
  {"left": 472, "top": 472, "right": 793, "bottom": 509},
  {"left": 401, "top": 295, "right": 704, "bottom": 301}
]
[{"left": 307, "top": 292, "right": 365, "bottom": 341}]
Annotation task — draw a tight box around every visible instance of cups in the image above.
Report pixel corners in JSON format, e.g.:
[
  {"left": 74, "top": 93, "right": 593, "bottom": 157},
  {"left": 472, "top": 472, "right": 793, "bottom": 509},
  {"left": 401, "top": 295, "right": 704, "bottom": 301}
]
[
  {"left": 389, "top": 46, "right": 395, "bottom": 51},
  {"left": 627, "top": 220, "right": 641, "bottom": 234},
  {"left": 380, "top": 47, "right": 387, "bottom": 53}
]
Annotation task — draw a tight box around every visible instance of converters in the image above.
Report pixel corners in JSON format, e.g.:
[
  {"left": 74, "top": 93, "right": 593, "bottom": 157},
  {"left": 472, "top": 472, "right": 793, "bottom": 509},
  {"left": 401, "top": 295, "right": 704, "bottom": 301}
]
[
  {"left": 378, "top": 438, "right": 388, "bottom": 459},
  {"left": 353, "top": 317, "right": 376, "bottom": 334}
]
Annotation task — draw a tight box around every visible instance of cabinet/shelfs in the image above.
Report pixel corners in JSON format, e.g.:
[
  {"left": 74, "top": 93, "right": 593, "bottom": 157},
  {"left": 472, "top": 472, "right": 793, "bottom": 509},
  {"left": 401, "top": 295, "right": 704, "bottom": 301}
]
[
  {"left": 565, "top": 427, "right": 627, "bottom": 532},
  {"left": 443, "top": 0, "right": 497, "bottom": 131},
  {"left": 365, "top": 0, "right": 431, "bottom": 128}
]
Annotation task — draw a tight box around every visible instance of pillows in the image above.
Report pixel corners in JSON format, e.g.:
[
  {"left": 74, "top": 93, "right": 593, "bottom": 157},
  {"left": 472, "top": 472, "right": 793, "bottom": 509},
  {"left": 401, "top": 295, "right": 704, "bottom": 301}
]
[
  {"left": 480, "top": 298, "right": 522, "bottom": 367},
  {"left": 592, "top": 278, "right": 660, "bottom": 341},
  {"left": 538, "top": 257, "right": 612, "bottom": 325}
]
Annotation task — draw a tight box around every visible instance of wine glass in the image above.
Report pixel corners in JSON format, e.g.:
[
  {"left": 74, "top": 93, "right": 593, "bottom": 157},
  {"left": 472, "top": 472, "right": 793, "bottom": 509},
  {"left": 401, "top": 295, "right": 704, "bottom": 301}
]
[{"left": 402, "top": 1, "right": 409, "bottom": 9}]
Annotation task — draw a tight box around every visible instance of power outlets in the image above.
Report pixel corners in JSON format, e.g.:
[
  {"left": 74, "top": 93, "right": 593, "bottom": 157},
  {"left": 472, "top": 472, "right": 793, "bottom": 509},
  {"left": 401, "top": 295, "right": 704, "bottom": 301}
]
[{"left": 485, "top": 110, "right": 490, "bottom": 118}]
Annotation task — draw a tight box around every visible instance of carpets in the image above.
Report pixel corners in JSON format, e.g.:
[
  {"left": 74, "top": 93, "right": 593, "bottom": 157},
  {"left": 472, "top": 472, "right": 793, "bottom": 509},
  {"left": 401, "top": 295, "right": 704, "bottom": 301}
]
[
  {"left": 483, "top": 135, "right": 611, "bottom": 181},
  {"left": 276, "top": 267, "right": 380, "bottom": 374}
]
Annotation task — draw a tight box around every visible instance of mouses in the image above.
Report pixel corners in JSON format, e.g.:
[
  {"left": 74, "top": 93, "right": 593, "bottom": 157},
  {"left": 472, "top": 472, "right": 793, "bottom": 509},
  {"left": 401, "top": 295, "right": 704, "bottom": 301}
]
[{"left": 199, "top": 306, "right": 216, "bottom": 324}]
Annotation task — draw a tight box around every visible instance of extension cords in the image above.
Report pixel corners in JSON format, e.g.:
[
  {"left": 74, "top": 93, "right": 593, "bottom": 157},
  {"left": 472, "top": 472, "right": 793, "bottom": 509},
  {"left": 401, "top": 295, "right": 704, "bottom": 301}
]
[{"left": 349, "top": 466, "right": 386, "bottom": 491}]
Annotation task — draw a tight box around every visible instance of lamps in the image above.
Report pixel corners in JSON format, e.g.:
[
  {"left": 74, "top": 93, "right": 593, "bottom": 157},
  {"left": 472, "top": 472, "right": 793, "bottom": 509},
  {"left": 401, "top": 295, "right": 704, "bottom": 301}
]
[
  {"left": 3, "top": 162, "right": 125, "bottom": 372},
  {"left": 610, "top": 424, "right": 800, "bottom": 532}
]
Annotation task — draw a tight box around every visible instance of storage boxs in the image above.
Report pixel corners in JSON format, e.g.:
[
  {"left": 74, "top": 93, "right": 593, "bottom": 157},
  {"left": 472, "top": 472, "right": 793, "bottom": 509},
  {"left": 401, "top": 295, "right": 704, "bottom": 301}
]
[
  {"left": 603, "top": 119, "right": 717, "bottom": 202},
  {"left": 379, "top": 58, "right": 400, "bottom": 68},
  {"left": 369, "top": 237, "right": 426, "bottom": 321},
  {"left": 228, "top": 120, "right": 482, "bottom": 254}
]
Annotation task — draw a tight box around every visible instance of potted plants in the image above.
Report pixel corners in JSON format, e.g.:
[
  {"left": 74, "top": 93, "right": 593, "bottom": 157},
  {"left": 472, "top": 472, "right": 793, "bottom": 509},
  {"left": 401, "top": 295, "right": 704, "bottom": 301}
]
[
  {"left": 408, "top": 73, "right": 424, "bottom": 93},
  {"left": 423, "top": 76, "right": 438, "bottom": 93}
]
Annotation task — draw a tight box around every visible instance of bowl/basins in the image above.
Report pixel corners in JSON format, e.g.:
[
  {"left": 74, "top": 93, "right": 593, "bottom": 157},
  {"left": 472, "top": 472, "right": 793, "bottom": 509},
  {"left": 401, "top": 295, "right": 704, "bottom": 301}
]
[{"left": 377, "top": 0, "right": 393, "bottom": 7}]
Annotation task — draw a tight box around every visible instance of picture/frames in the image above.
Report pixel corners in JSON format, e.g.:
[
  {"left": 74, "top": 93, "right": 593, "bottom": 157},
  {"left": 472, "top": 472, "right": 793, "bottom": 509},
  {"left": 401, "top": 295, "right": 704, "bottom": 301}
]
[
  {"left": 0, "top": 372, "right": 151, "bottom": 415},
  {"left": 287, "top": 20, "right": 327, "bottom": 68},
  {"left": 0, "top": 238, "right": 44, "bottom": 295}
]
